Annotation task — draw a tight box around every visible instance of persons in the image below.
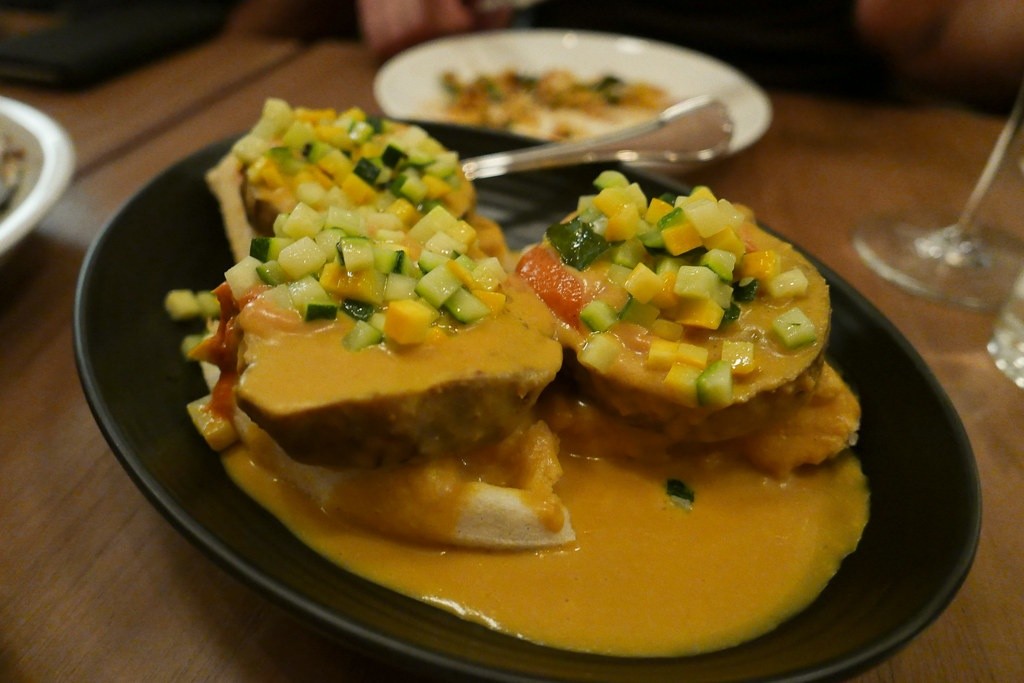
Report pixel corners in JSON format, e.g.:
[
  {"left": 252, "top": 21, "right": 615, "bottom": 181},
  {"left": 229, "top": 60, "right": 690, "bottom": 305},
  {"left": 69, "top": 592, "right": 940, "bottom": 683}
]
[
  {"left": 358, "top": 0, "right": 1024, "bottom": 118},
  {"left": 0, "top": 0, "right": 227, "bottom": 93}
]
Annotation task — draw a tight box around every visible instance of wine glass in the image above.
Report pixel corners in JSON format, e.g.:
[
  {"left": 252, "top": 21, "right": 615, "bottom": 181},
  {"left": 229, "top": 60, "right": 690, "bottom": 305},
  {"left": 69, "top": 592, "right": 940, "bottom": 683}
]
[{"left": 850, "top": 82, "right": 1024, "bottom": 310}]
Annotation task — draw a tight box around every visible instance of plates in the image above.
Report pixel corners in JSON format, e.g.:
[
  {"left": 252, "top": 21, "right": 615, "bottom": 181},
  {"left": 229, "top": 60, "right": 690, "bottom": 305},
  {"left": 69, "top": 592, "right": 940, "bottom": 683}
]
[
  {"left": 75, "top": 116, "right": 983, "bottom": 683},
  {"left": 373, "top": 28, "right": 773, "bottom": 163},
  {"left": 0, "top": 95, "right": 78, "bottom": 261}
]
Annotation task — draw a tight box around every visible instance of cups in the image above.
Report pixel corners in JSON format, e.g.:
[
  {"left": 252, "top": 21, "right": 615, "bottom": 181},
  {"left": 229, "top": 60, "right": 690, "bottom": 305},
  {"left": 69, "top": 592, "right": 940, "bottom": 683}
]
[{"left": 986, "top": 263, "right": 1024, "bottom": 387}]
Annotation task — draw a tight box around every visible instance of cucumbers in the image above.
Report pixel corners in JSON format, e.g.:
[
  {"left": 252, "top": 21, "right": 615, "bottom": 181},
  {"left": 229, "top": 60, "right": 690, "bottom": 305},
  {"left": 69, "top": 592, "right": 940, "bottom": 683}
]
[{"left": 166, "top": 96, "right": 819, "bottom": 511}]
